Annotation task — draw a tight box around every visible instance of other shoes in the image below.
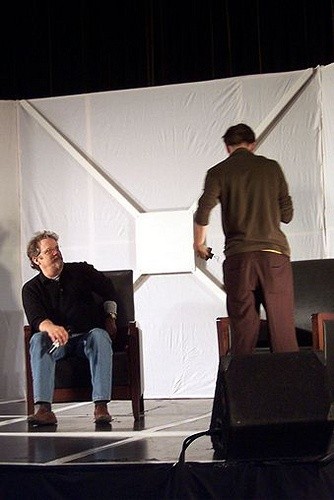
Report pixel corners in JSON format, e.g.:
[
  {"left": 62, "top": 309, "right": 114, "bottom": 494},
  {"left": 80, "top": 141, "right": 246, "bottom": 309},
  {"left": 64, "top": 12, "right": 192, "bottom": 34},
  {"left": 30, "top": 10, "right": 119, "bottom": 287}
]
[
  {"left": 27, "top": 407, "right": 58, "bottom": 425},
  {"left": 94, "top": 405, "right": 112, "bottom": 423}
]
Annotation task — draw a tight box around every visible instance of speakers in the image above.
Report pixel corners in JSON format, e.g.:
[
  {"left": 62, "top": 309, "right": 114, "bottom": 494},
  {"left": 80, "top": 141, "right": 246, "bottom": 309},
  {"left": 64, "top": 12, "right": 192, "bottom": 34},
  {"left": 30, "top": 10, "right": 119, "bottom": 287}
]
[{"left": 207, "top": 349, "right": 332, "bottom": 459}]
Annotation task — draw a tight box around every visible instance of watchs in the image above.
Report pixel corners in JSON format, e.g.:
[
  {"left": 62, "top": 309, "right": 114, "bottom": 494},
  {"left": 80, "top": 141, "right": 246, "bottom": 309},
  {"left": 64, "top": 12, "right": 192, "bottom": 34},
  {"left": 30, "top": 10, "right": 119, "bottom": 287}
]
[{"left": 105, "top": 312, "right": 117, "bottom": 320}]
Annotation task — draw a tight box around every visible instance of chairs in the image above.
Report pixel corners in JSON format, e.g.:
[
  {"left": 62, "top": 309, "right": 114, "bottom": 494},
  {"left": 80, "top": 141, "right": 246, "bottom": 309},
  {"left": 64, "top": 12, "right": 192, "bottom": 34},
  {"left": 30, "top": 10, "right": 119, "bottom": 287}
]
[
  {"left": 216, "top": 256, "right": 334, "bottom": 372},
  {"left": 25, "top": 270, "right": 147, "bottom": 416}
]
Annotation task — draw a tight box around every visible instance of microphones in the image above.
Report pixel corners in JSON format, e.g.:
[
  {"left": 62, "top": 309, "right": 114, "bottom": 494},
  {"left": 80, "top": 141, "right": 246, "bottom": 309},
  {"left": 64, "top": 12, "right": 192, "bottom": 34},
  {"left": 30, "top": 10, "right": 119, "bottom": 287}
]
[{"left": 50, "top": 326, "right": 73, "bottom": 355}]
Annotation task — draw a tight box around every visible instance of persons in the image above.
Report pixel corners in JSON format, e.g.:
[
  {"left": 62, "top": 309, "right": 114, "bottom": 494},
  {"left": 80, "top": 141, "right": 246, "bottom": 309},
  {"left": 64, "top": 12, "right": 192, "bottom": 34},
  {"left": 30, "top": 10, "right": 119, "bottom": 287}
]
[
  {"left": 21, "top": 229, "right": 120, "bottom": 426},
  {"left": 192, "top": 122, "right": 300, "bottom": 358}
]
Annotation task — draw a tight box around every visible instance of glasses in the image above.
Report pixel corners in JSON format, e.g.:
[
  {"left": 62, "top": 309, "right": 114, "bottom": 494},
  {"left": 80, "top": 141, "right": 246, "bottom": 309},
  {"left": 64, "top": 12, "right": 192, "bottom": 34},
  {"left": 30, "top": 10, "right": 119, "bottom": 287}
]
[{"left": 37, "top": 245, "right": 61, "bottom": 257}]
